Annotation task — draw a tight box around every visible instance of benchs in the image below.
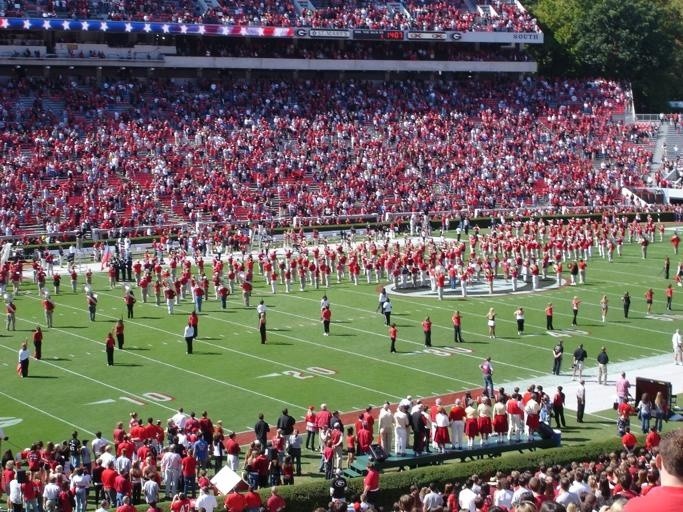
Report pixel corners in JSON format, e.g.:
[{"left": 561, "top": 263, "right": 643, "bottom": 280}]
[
  {"left": 250, "top": 121, "right": 448, "bottom": 217},
  {"left": 16, "top": 88, "right": 216, "bottom": 236},
  {"left": 532, "top": 115, "right": 681, "bottom": 206}
]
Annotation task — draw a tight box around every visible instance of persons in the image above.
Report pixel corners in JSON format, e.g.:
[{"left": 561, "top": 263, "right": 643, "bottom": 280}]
[{"left": 0, "top": 0, "right": 682, "bottom": 512}]
